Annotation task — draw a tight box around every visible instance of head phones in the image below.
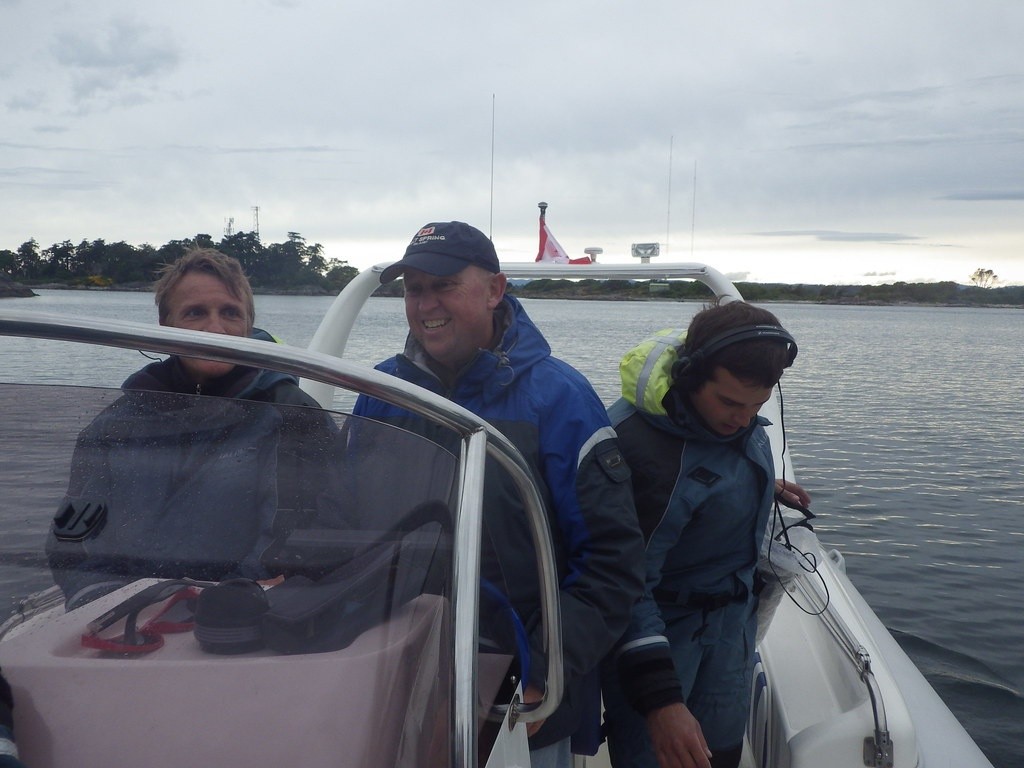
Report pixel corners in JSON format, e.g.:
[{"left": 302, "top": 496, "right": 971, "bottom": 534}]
[{"left": 669, "top": 325, "right": 799, "bottom": 394}]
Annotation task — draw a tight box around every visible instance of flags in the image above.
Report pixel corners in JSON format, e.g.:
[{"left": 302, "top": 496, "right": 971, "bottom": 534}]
[{"left": 536, "top": 222, "right": 591, "bottom": 265}]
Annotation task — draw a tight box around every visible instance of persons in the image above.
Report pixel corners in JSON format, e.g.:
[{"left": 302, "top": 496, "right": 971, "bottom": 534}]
[{"left": 48, "top": 221, "right": 810, "bottom": 768}]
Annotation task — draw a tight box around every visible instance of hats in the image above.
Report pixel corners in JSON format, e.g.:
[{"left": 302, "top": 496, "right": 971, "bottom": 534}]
[{"left": 379, "top": 221, "right": 500, "bottom": 284}]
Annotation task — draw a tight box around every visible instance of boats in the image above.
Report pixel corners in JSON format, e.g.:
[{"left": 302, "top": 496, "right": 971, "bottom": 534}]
[{"left": 0, "top": 262, "right": 997, "bottom": 768}]
